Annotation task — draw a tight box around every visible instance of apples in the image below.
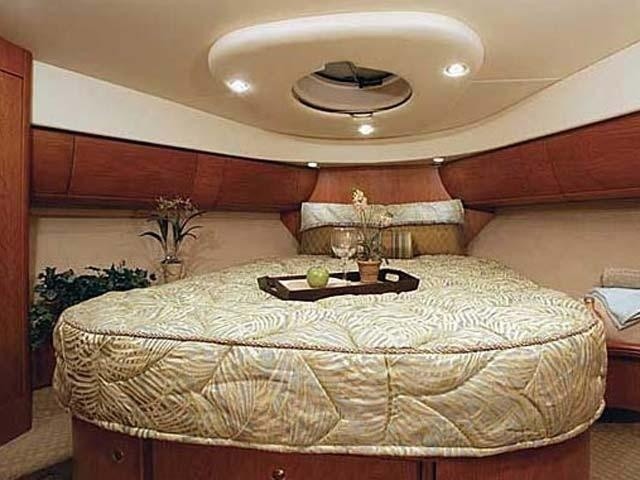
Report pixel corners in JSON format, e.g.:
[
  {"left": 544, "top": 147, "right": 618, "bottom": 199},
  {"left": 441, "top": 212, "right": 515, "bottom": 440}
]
[{"left": 306, "top": 266, "right": 329, "bottom": 287}]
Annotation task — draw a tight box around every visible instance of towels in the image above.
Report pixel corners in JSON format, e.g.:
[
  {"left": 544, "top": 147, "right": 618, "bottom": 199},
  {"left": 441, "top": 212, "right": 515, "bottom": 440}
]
[{"left": 589, "top": 285, "right": 640, "bottom": 331}]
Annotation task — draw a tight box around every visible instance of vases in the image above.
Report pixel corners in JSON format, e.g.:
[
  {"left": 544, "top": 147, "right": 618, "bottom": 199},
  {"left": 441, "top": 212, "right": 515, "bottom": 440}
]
[
  {"left": 356, "top": 260, "right": 381, "bottom": 284},
  {"left": 161, "top": 258, "right": 186, "bottom": 283}
]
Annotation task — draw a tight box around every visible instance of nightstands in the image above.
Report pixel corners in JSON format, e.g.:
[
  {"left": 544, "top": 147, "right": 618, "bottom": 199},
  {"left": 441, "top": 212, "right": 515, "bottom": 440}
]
[{"left": 603, "top": 340, "right": 640, "bottom": 416}]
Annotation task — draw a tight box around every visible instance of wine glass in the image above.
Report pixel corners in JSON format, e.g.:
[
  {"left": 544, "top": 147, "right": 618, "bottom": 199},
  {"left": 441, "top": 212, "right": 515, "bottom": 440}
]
[{"left": 330, "top": 220, "right": 359, "bottom": 280}]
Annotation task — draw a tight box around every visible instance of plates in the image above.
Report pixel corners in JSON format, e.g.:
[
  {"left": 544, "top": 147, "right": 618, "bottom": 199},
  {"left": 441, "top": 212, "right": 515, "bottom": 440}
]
[{"left": 275, "top": 276, "right": 350, "bottom": 293}]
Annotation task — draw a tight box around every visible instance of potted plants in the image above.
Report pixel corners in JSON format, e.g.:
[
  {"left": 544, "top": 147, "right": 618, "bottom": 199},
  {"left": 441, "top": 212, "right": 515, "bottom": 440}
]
[{"left": 29, "top": 260, "right": 151, "bottom": 390}]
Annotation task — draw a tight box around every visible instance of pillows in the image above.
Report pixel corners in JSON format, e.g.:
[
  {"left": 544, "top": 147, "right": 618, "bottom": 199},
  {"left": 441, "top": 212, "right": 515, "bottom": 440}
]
[{"left": 297, "top": 200, "right": 467, "bottom": 256}]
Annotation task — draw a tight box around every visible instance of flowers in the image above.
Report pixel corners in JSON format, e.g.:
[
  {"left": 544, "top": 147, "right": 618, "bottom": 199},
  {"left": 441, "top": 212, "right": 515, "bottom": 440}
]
[
  {"left": 138, "top": 195, "right": 208, "bottom": 264},
  {"left": 347, "top": 187, "right": 394, "bottom": 266}
]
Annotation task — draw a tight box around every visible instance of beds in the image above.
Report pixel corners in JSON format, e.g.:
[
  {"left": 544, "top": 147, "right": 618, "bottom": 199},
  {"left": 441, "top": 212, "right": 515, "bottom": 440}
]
[{"left": 52, "top": 197, "right": 609, "bottom": 480}]
